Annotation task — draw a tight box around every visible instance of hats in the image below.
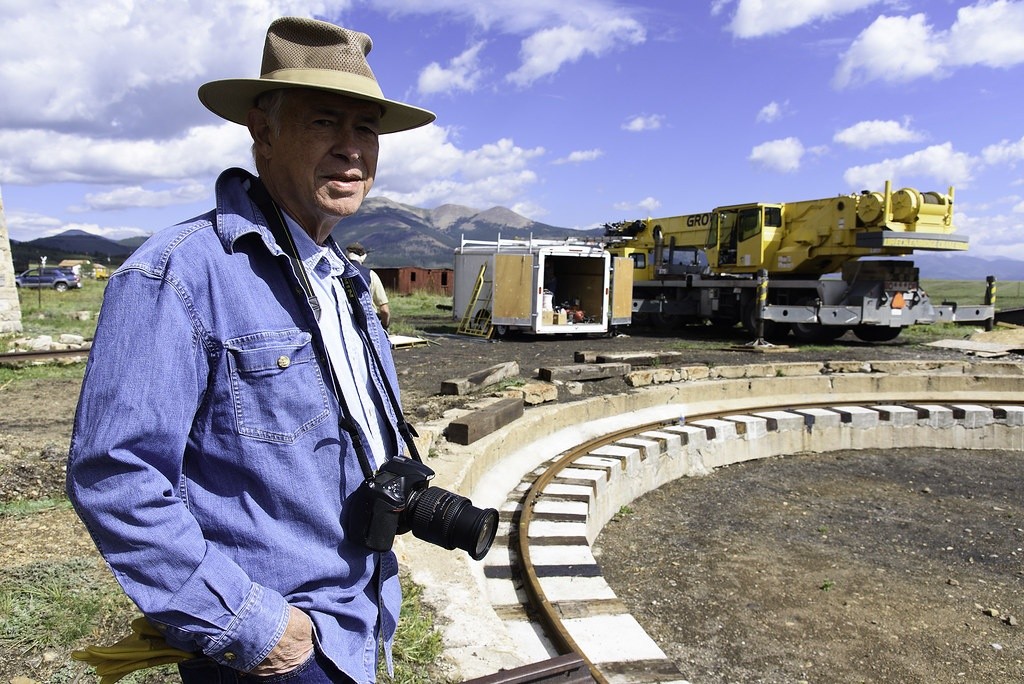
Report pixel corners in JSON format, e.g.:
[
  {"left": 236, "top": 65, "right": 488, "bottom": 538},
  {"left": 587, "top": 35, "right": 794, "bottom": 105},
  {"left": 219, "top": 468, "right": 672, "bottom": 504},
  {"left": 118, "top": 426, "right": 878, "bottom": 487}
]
[{"left": 198, "top": 16, "right": 435, "bottom": 135}]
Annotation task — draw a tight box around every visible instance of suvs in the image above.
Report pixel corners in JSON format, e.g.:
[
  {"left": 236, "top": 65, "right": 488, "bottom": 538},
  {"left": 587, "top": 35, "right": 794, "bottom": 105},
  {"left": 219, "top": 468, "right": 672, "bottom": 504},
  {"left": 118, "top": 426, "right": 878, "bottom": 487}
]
[{"left": 15, "top": 265, "right": 82, "bottom": 293}]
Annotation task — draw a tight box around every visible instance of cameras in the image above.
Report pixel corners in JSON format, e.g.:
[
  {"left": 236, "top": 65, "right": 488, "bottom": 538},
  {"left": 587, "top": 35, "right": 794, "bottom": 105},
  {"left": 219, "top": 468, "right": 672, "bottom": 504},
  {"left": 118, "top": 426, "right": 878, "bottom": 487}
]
[{"left": 342, "top": 455, "right": 499, "bottom": 560}]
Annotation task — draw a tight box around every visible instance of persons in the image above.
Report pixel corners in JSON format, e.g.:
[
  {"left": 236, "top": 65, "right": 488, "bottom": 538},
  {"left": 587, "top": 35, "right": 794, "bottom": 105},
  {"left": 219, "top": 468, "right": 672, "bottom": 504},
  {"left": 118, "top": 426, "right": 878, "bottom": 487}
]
[{"left": 67, "top": 17, "right": 437, "bottom": 684}]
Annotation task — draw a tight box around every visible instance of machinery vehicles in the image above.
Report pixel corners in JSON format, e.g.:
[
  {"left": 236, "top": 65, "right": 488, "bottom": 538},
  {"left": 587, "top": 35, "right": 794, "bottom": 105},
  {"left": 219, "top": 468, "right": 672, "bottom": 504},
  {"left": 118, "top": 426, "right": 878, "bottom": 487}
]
[{"left": 600, "top": 181, "right": 995, "bottom": 343}]
